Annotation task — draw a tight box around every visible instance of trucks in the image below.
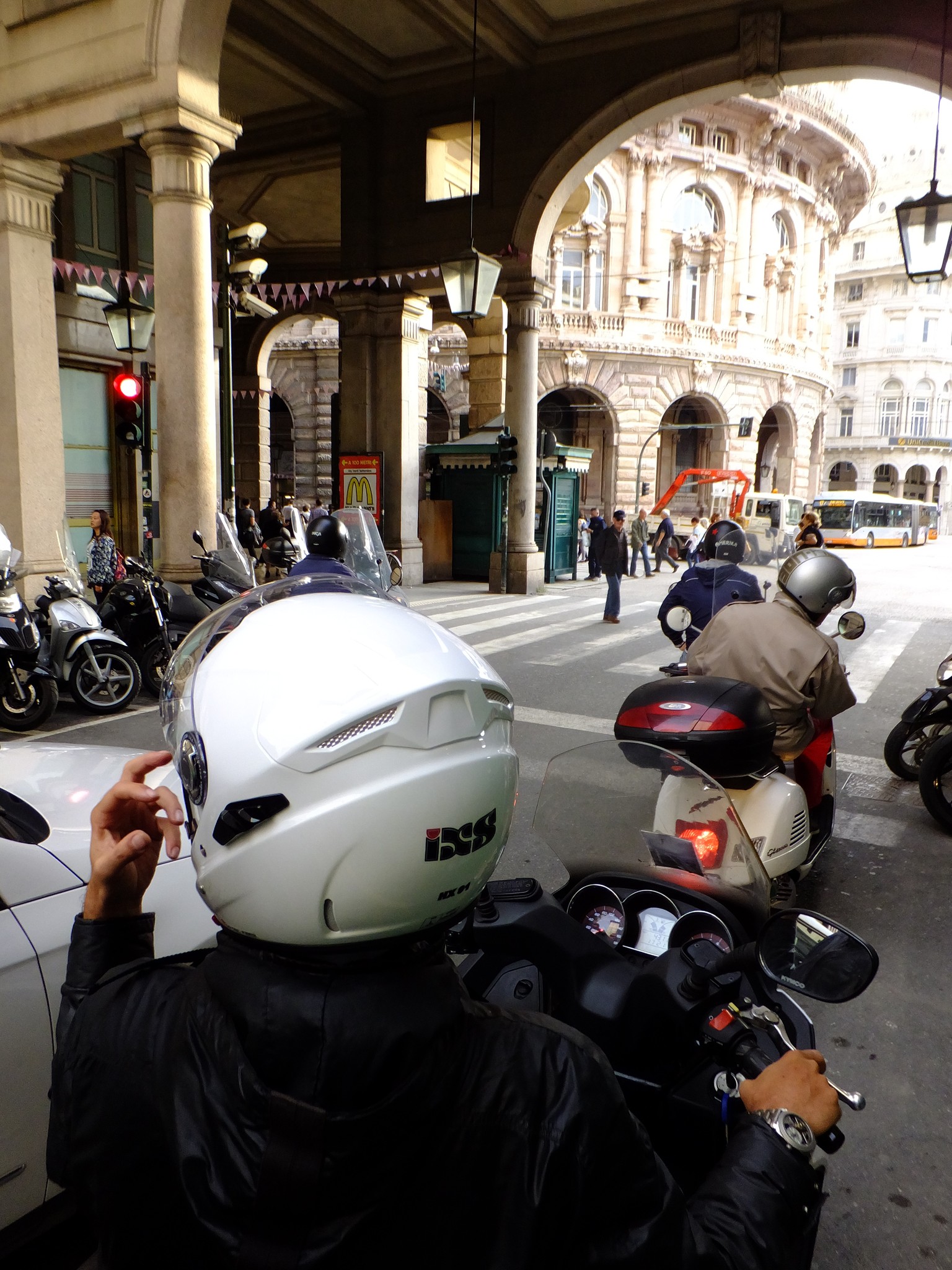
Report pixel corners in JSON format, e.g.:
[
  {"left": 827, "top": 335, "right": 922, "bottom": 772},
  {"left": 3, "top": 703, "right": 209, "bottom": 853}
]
[{"left": 625, "top": 466, "right": 804, "bottom": 568}]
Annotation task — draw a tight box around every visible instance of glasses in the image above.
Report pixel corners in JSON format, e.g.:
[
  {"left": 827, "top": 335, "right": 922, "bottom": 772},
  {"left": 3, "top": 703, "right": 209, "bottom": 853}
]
[{"left": 615, "top": 517, "right": 625, "bottom": 521}]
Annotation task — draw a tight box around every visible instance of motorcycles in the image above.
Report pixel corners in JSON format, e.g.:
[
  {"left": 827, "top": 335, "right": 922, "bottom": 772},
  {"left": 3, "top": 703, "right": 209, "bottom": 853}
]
[
  {"left": 443, "top": 736, "right": 883, "bottom": 1268},
  {"left": 612, "top": 606, "right": 871, "bottom": 913},
  {"left": 883, "top": 650, "right": 952, "bottom": 838},
  {"left": 1, "top": 500, "right": 411, "bottom": 733}
]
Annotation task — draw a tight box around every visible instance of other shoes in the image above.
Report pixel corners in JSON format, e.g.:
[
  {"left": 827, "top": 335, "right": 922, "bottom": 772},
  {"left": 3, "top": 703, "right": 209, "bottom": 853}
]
[
  {"left": 650, "top": 569, "right": 661, "bottom": 572},
  {"left": 265, "top": 572, "right": 271, "bottom": 578},
  {"left": 646, "top": 574, "right": 655, "bottom": 578},
  {"left": 259, "top": 563, "right": 266, "bottom": 567},
  {"left": 630, "top": 575, "right": 638, "bottom": 578},
  {"left": 585, "top": 559, "right": 588, "bottom": 562},
  {"left": 275, "top": 573, "right": 281, "bottom": 577},
  {"left": 583, "top": 575, "right": 594, "bottom": 581},
  {"left": 577, "top": 560, "right": 584, "bottom": 564},
  {"left": 592, "top": 575, "right": 602, "bottom": 581},
  {"left": 672, "top": 564, "right": 679, "bottom": 573},
  {"left": 255, "top": 565, "right": 260, "bottom": 569},
  {"left": 808, "top": 795, "right": 833, "bottom": 833},
  {"left": 603, "top": 613, "right": 621, "bottom": 624}
]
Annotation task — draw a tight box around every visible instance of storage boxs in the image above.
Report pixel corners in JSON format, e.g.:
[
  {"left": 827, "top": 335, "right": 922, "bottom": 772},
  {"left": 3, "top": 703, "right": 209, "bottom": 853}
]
[{"left": 612, "top": 676, "right": 778, "bottom": 774}]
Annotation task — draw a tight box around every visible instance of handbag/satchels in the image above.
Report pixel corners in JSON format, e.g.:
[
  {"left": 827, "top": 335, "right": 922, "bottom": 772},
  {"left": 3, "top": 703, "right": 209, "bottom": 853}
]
[{"left": 697, "top": 541, "right": 705, "bottom": 554}]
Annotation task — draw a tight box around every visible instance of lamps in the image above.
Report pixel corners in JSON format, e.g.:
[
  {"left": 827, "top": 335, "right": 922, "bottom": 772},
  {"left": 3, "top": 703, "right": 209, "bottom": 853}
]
[
  {"left": 429, "top": 339, "right": 440, "bottom": 353},
  {"left": 894, "top": 0, "right": 951, "bottom": 284},
  {"left": 890, "top": 467, "right": 896, "bottom": 490},
  {"left": 101, "top": 146, "right": 155, "bottom": 355},
  {"left": 435, "top": 1, "right": 504, "bottom": 330}
]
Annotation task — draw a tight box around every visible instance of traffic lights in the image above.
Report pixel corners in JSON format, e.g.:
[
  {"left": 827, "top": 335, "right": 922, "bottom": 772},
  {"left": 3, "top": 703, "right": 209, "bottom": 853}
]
[
  {"left": 496, "top": 432, "right": 519, "bottom": 477},
  {"left": 112, "top": 373, "right": 146, "bottom": 449},
  {"left": 641, "top": 482, "right": 650, "bottom": 496}
]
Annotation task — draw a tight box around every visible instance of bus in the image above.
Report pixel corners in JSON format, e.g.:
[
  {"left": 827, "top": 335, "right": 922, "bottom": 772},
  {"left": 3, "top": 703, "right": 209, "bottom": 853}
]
[
  {"left": 925, "top": 501, "right": 941, "bottom": 540},
  {"left": 803, "top": 490, "right": 930, "bottom": 546}
]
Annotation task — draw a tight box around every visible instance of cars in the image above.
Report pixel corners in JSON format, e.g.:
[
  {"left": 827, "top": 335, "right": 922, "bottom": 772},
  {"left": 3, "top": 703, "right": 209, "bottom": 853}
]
[{"left": 0, "top": 738, "right": 227, "bottom": 1241}]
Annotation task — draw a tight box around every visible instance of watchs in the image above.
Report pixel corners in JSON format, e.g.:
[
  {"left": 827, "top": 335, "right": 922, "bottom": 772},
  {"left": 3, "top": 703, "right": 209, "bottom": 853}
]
[{"left": 755, "top": 1106, "right": 817, "bottom": 1157}]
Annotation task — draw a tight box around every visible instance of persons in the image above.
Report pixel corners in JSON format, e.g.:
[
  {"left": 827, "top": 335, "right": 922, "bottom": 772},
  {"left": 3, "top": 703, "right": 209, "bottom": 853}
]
[
  {"left": 651, "top": 508, "right": 680, "bottom": 573},
  {"left": 235, "top": 496, "right": 260, "bottom": 570},
  {"left": 265, "top": 510, "right": 281, "bottom": 578},
  {"left": 84, "top": 508, "right": 119, "bottom": 605},
  {"left": 684, "top": 511, "right": 825, "bottom": 569},
  {"left": 628, "top": 509, "right": 656, "bottom": 579},
  {"left": 285, "top": 514, "right": 359, "bottom": 597},
  {"left": 43, "top": 572, "right": 844, "bottom": 1268},
  {"left": 257, "top": 499, "right": 277, "bottom": 567},
  {"left": 583, "top": 507, "right": 607, "bottom": 581},
  {"left": 656, "top": 519, "right": 765, "bottom": 663},
  {"left": 686, "top": 547, "right": 857, "bottom": 839},
  {"left": 535, "top": 503, "right": 591, "bottom": 563},
  {"left": 277, "top": 493, "right": 333, "bottom": 537},
  {"left": 594, "top": 510, "right": 629, "bottom": 624}
]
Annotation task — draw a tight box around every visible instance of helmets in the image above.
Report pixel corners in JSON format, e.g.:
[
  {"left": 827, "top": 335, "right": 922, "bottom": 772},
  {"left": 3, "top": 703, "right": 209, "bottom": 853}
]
[
  {"left": 158, "top": 577, "right": 520, "bottom": 949},
  {"left": 306, "top": 515, "right": 351, "bottom": 563},
  {"left": 704, "top": 520, "right": 746, "bottom": 562},
  {"left": 777, "top": 547, "right": 857, "bottom": 615}
]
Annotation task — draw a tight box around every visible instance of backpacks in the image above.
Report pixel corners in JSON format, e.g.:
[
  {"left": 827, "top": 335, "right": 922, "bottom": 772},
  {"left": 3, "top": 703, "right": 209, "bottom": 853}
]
[
  {"left": 114, "top": 546, "right": 127, "bottom": 585},
  {"left": 579, "top": 522, "right": 588, "bottom": 536}
]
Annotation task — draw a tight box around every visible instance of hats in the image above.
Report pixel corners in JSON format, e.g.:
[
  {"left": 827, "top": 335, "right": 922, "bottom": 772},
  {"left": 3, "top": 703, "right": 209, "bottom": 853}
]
[{"left": 613, "top": 510, "right": 626, "bottom": 518}]
